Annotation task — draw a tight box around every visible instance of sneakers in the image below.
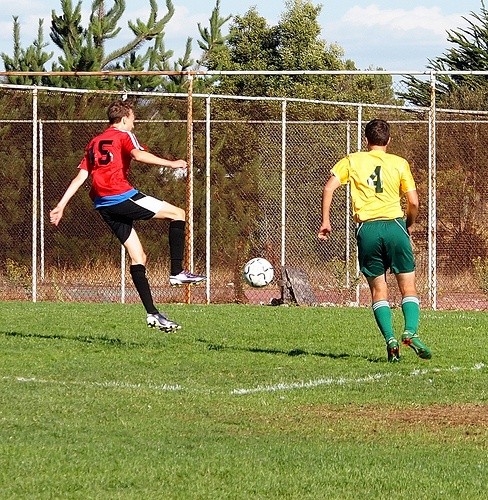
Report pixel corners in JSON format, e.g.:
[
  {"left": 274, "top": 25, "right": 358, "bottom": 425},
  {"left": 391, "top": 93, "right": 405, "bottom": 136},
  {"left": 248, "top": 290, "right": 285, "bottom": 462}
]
[
  {"left": 386, "top": 342, "right": 400, "bottom": 363},
  {"left": 168, "top": 269, "right": 205, "bottom": 288},
  {"left": 402, "top": 332, "right": 432, "bottom": 359},
  {"left": 146, "top": 313, "right": 180, "bottom": 333}
]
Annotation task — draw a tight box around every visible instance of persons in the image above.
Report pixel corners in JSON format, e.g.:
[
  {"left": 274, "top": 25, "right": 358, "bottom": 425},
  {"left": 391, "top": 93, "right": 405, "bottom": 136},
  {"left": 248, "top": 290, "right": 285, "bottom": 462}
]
[
  {"left": 48, "top": 102, "right": 210, "bottom": 333},
  {"left": 318, "top": 119, "right": 434, "bottom": 363}
]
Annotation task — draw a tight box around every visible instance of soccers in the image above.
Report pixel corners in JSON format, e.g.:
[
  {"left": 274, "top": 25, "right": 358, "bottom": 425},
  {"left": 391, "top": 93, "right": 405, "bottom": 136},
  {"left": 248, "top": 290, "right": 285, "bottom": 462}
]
[{"left": 243, "top": 257, "right": 274, "bottom": 287}]
